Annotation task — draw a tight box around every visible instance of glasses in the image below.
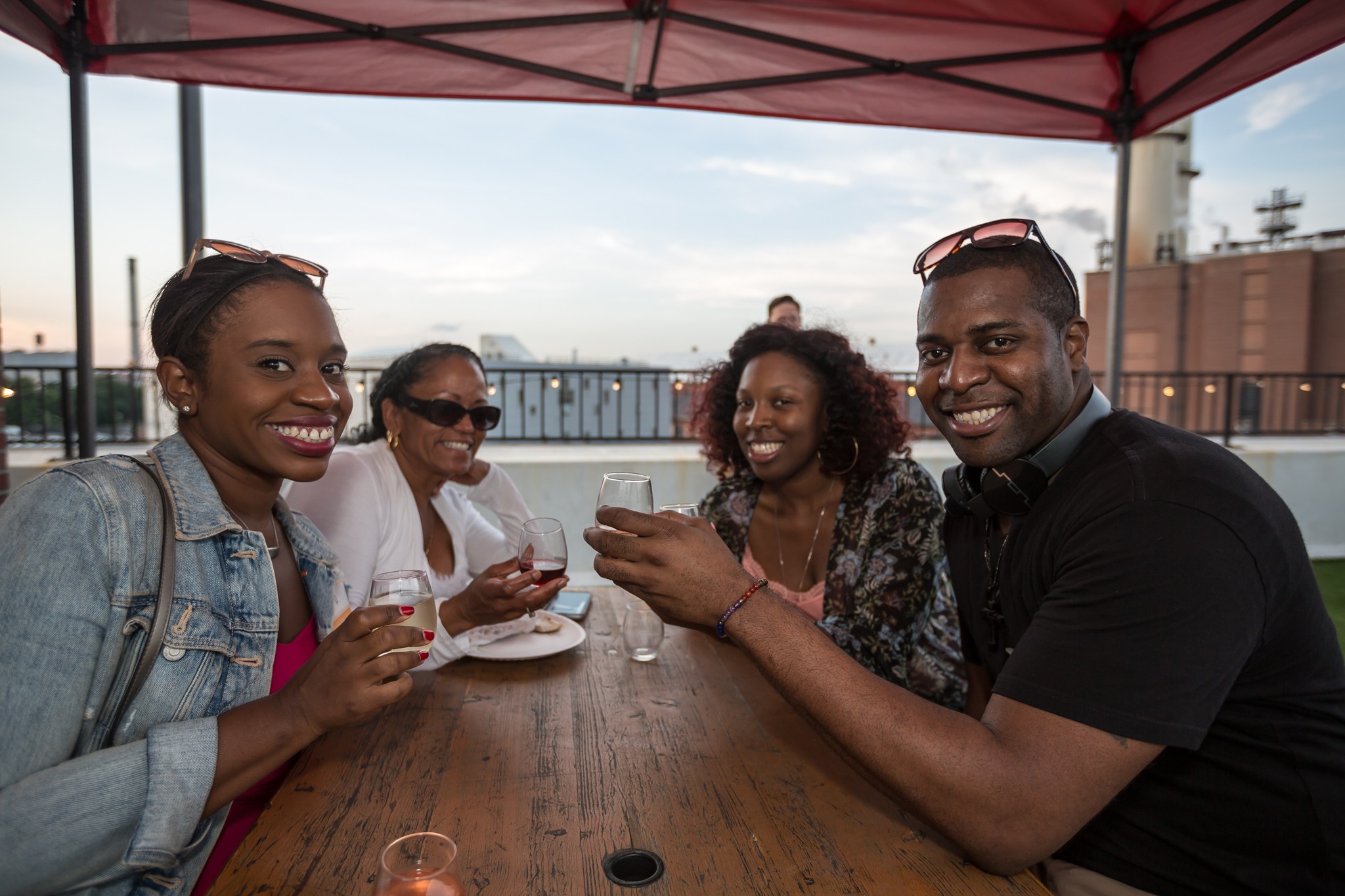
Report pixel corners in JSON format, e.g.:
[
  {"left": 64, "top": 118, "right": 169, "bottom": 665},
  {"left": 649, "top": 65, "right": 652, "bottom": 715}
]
[
  {"left": 394, "top": 392, "right": 502, "bottom": 432},
  {"left": 912, "top": 218, "right": 1080, "bottom": 316},
  {"left": 182, "top": 237, "right": 329, "bottom": 292}
]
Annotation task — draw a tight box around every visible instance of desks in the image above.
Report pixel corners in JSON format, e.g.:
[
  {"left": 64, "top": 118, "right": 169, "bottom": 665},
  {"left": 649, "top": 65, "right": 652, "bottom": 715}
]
[{"left": 189, "top": 586, "right": 1057, "bottom": 896}]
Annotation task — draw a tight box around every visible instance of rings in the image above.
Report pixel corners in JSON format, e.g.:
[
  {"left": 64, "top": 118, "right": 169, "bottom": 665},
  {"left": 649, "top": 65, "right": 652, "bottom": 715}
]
[{"left": 524, "top": 607, "right": 534, "bottom": 617}]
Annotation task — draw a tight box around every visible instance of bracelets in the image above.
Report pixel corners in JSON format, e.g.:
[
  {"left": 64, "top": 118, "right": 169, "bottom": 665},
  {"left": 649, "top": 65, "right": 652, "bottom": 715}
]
[{"left": 710, "top": 574, "right": 771, "bottom": 638}]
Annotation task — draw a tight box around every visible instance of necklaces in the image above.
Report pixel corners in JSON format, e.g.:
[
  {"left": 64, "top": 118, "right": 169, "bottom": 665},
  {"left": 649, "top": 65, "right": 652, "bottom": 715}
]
[
  {"left": 424, "top": 519, "right": 434, "bottom": 555},
  {"left": 218, "top": 499, "right": 281, "bottom": 560},
  {"left": 773, "top": 481, "right": 837, "bottom": 594}
]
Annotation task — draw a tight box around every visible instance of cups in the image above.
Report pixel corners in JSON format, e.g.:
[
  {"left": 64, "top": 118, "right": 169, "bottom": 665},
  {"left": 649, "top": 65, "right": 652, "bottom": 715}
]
[
  {"left": 368, "top": 569, "right": 437, "bottom": 656},
  {"left": 372, "top": 831, "right": 469, "bottom": 896},
  {"left": 595, "top": 471, "right": 655, "bottom": 538},
  {"left": 518, "top": 517, "right": 568, "bottom": 588},
  {"left": 621, "top": 600, "right": 665, "bottom": 662},
  {"left": 659, "top": 504, "right": 703, "bottom": 517}
]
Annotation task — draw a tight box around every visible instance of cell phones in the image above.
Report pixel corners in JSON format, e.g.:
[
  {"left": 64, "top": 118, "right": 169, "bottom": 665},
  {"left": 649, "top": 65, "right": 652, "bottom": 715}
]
[{"left": 543, "top": 590, "right": 591, "bottom": 621}]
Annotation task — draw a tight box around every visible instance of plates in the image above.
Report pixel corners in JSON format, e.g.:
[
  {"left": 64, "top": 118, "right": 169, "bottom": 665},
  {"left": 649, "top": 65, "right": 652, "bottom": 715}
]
[{"left": 465, "top": 609, "right": 587, "bottom": 662}]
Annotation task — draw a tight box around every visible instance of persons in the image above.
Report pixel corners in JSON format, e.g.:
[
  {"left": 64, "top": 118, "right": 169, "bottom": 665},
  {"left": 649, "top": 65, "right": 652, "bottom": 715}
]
[
  {"left": 283, "top": 342, "right": 572, "bottom": 676},
  {"left": 583, "top": 215, "right": 1345, "bottom": 895},
  {"left": 763, "top": 294, "right": 802, "bottom": 333},
  {"left": 686, "top": 323, "right": 972, "bottom": 712},
  {"left": 1, "top": 236, "right": 435, "bottom": 896}
]
